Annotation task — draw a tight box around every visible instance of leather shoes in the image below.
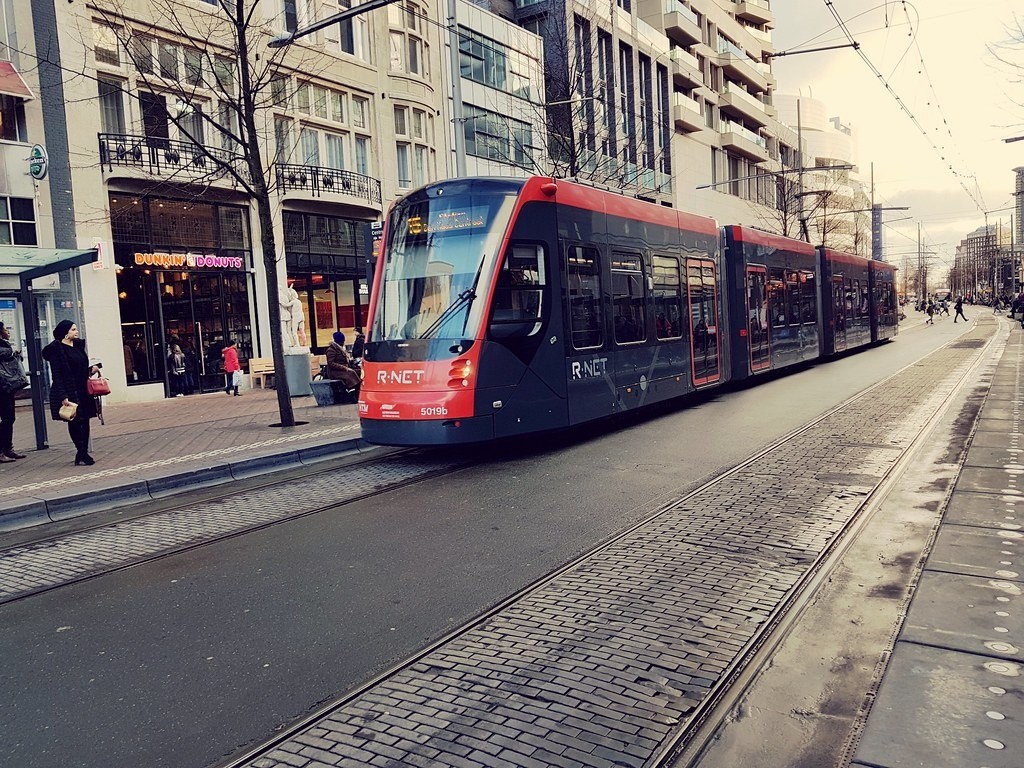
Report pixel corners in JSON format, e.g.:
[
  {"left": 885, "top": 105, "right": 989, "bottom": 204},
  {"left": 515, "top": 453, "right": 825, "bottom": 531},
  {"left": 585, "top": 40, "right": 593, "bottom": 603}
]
[
  {"left": 0, "top": 452, "right": 16, "bottom": 463},
  {"left": 4, "top": 449, "right": 27, "bottom": 459}
]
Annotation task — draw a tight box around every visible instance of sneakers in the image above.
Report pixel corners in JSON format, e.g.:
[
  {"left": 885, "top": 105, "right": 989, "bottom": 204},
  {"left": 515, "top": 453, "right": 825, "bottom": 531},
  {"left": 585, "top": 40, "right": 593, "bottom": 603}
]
[
  {"left": 177, "top": 393, "right": 184, "bottom": 398},
  {"left": 234, "top": 386, "right": 242, "bottom": 396},
  {"left": 223, "top": 385, "right": 231, "bottom": 395}
]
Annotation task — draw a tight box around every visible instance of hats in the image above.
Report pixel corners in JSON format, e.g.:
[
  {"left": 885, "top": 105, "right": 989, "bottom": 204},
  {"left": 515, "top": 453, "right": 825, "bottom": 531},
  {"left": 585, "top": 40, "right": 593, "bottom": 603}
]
[
  {"left": 332, "top": 331, "right": 346, "bottom": 344},
  {"left": 52, "top": 319, "right": 76, "bottom": 341}
]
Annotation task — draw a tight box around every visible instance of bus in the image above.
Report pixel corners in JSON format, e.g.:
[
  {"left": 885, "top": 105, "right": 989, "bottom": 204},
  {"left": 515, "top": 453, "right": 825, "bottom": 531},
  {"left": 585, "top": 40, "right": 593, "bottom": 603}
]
[
  {"left": 934, "top": 288, "right": 953, "bottom": 307},
  {"left": 357, "top": 175, "right": 900, "bottom": 450}
]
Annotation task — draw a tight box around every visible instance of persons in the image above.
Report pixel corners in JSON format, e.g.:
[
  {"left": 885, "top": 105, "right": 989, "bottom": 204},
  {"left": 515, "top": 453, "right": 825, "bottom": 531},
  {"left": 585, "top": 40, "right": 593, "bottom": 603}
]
[
  {"left": 41, "top": 319, "right": 100, "bottom": 465},
  {"left": 657, "top": 312, "right": 761, "bottom": 355},
  {"left": 125, "top": 284, "right": 1024, "bottom": 398},
  {"left": 279, "top": 289, "right": 296, "bottom": 346},
  {"left": 0, "top": 323, "right": 27, "bottom": 462},
  {"left": 279, "top": 288, "right": 307, "bottom": 348}
]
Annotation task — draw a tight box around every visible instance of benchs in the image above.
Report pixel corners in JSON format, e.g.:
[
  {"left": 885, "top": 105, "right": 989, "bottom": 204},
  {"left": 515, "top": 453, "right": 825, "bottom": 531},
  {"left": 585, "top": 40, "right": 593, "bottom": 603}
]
[{"left": 249, "top": 354, "right": 320, "bottom": 389}]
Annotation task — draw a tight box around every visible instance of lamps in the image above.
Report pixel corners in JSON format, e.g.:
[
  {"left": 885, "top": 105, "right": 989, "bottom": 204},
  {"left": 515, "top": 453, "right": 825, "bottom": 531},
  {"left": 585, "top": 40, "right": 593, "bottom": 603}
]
[
  {"left": 324, "top": 276, "right": 334, "bottom": 294},
  {"left": 300, "top": 278, "right": 324, "bottom": 300}
]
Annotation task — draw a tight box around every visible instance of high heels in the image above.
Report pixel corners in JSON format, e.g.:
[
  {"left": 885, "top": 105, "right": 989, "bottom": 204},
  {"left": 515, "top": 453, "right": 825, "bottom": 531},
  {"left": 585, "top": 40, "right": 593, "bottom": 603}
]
[{"left": 68, "top": 421, "right": 96, "bottom": 466}]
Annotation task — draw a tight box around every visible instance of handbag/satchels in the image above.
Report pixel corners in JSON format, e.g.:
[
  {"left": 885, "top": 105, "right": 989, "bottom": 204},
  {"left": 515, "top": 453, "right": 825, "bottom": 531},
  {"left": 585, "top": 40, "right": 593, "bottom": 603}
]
[
  {"left": 0, "top": 353, "right": 30, "bottom": 392},
  {"left": 85, "top": 370, "right": 111, "bottom": 398}
]
